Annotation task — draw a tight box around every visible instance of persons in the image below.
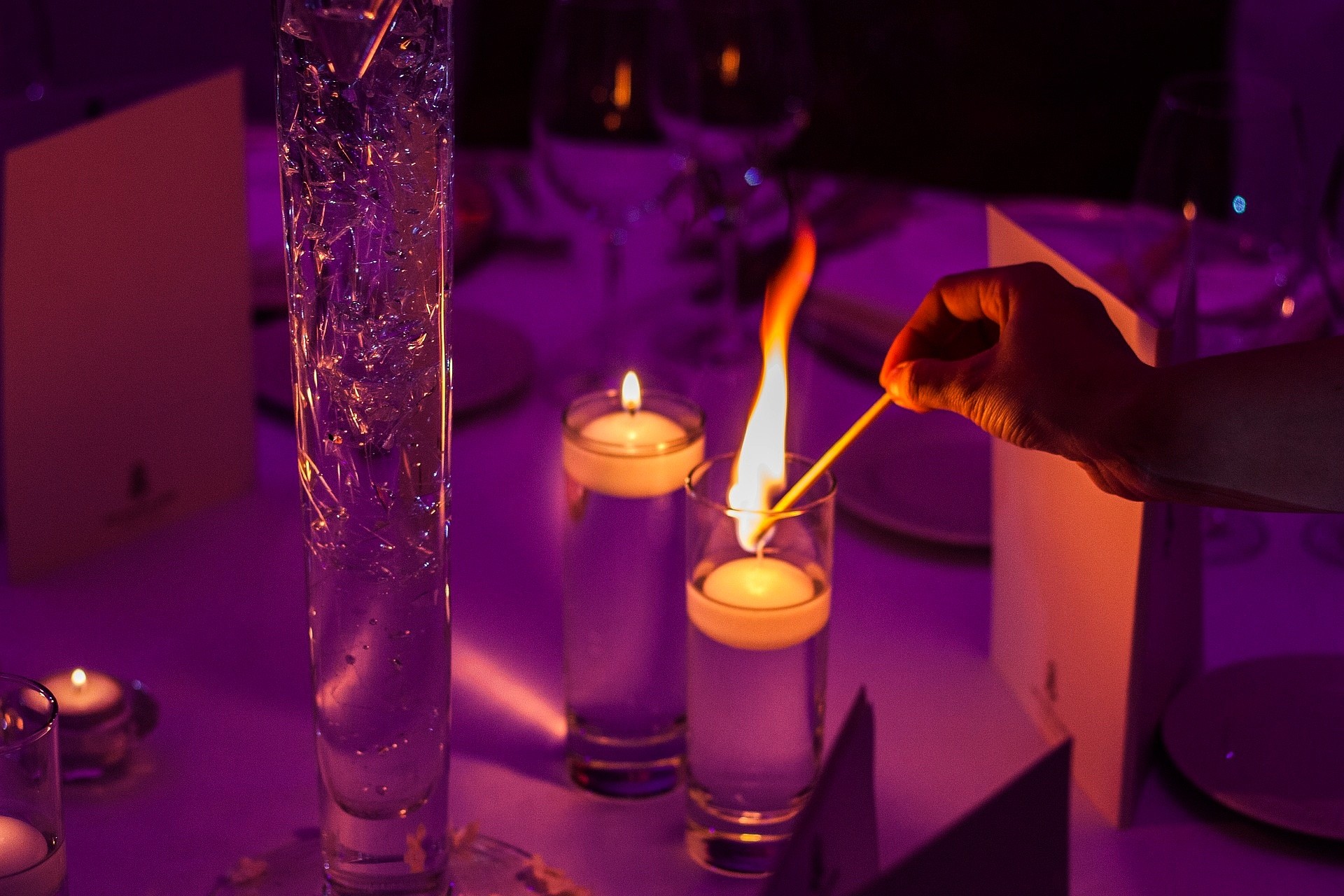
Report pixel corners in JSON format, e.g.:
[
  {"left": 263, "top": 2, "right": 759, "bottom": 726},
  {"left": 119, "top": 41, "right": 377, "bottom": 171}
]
[{"left": 881, "top": 261, "right": 1344, "bottom": 513}]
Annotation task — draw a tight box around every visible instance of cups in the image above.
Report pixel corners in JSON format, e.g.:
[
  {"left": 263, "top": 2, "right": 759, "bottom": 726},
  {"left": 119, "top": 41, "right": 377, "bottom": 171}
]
[
  {"left": 683, "top": 445, "right": 842, "bottom": 881},
  {"left": 552, "top": 387, "right": 710, "bottom": 799},
  {"left": 0, "top": 668, "right": 71, "bottom": 896}
]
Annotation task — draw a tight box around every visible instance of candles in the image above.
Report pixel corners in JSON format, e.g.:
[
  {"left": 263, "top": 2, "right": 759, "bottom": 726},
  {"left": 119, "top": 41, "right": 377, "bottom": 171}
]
[
  {"left": 17, "top": 666, "right": 167, "bottom": 789},
  {"left": 557, "top": 370, "right": 839, "bottom": 877}
]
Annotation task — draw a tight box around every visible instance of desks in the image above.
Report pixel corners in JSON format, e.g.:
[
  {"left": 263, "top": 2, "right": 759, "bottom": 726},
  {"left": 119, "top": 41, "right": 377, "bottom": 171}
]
[{"left": 0, "top": 80, "right": 1344, "bottom": 896}]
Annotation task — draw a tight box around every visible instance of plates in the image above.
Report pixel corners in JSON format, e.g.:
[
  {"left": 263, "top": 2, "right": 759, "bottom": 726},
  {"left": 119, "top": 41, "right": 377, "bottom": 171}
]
[
  {"left": 833, "top": 401, "right": 993, "bottom": 545},
  {"left": 1164, "top": 649, "right": 1344, "bottom": 838}
]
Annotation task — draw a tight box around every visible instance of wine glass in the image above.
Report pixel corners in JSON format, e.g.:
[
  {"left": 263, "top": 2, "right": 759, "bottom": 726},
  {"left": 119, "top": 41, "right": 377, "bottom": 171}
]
[{"left": 527, "top": 1, "right": 811, "bottom": 432}]
